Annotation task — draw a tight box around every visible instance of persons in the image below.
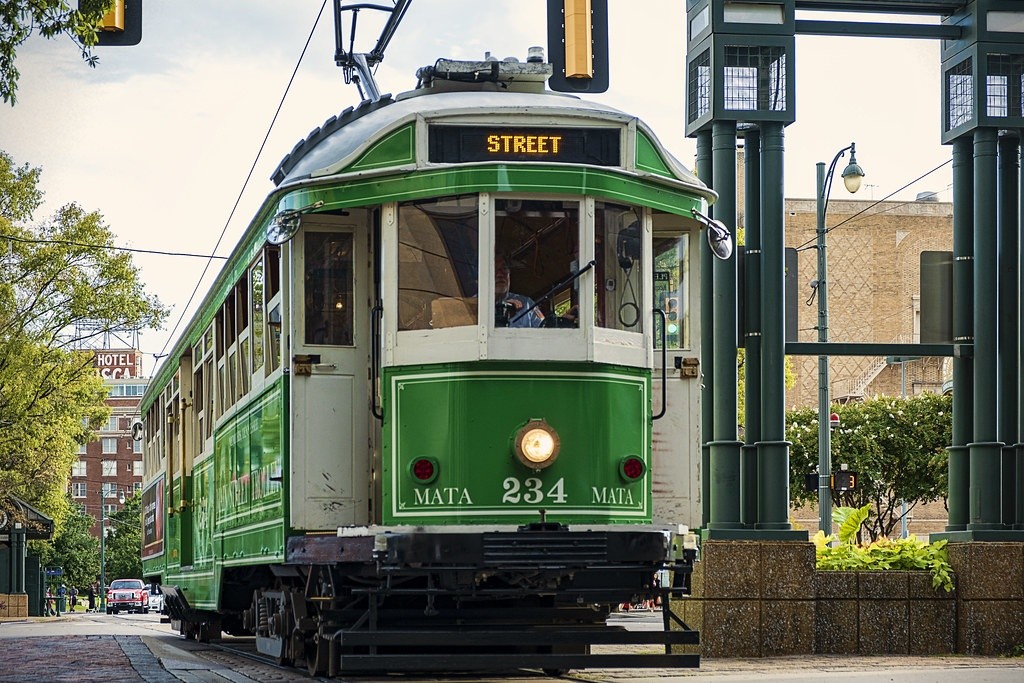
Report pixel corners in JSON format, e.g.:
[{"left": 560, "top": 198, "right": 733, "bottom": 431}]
[
  {"left": 469, "top": 252, "right": 580, "bottom": 328},
  {"left": 69, "top": 585, "right": 78, "bottom": 612}
]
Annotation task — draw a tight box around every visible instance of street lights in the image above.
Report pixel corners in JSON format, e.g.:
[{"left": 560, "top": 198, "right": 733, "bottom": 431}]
[
  {"left": 99, "top": 489, "right": 126, "bottom": 613},
  {"left": 815, "top": 141, "right": 866, "bottom": 550}
]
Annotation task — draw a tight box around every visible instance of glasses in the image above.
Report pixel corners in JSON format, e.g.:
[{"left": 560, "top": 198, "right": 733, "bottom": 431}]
[{"left": 495, "top": 266, "right": 511, "bottom": 274}]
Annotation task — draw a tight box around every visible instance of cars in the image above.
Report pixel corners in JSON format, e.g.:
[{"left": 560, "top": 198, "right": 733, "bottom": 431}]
[
  {"left": 145, "top": 583, "right": 166, "bottom": 613},
  {"left": 106, "top": 578, "right": 150, "bottom": 614}
]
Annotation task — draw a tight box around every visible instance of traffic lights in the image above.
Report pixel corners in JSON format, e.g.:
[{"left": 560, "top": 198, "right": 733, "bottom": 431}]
[{"left": 834, "top": 471, "right": 859, "bottom": 491}]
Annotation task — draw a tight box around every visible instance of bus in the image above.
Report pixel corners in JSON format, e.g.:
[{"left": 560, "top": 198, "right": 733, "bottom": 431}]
[{"left": 134, "top": 0, "right": 736, "bottom": 683}]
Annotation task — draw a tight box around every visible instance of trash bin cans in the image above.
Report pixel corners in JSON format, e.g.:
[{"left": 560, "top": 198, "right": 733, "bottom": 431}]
[{"left": 57, "top": 597, "right": 67, "bottom": 612}]
